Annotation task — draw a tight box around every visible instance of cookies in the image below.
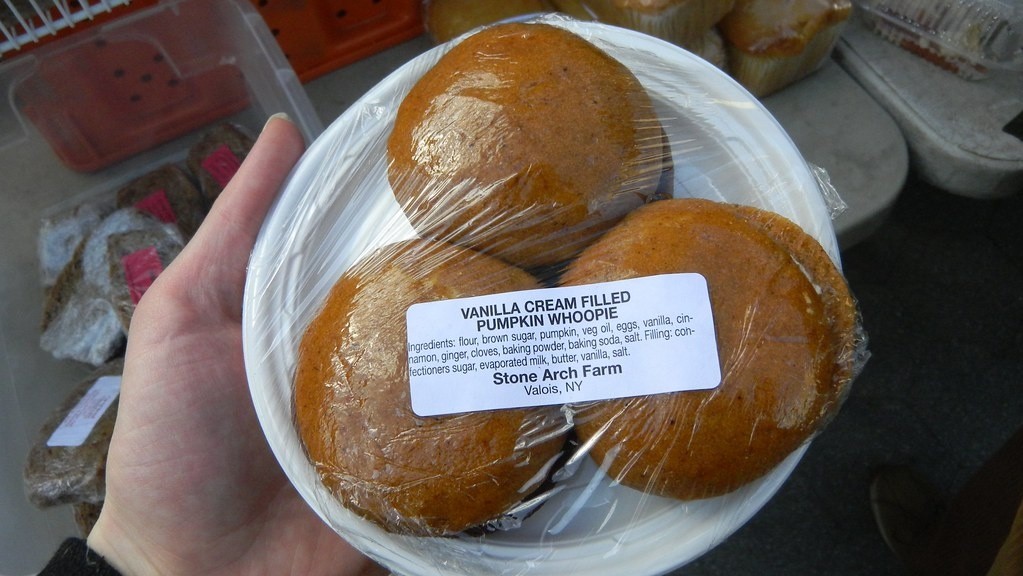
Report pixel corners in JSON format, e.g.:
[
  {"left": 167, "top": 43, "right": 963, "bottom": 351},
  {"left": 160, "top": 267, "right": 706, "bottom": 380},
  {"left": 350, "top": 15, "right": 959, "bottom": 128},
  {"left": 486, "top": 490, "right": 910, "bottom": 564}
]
[{"left": 23, "top": 123, "right": 257, "bottom": 509}]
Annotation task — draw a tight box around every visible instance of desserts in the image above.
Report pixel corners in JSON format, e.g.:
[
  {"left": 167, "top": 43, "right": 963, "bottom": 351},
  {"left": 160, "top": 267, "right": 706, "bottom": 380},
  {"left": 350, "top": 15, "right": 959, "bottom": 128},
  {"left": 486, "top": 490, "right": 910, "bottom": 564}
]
[{"left": 292, "top": 0, "right": 1023, "bottom": 536}]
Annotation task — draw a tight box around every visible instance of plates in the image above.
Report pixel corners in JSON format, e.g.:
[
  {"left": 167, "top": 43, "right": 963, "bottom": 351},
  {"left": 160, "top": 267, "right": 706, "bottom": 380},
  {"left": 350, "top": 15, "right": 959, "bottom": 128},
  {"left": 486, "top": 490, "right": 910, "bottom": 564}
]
[{"left": 241, "top": 14, "right": 842, "bottom": 576}]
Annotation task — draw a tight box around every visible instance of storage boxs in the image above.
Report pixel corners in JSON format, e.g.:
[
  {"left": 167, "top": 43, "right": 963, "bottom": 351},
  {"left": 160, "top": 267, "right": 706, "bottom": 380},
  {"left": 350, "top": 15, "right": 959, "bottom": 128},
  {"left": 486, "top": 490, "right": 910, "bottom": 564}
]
[
  {"left": 0, "top": 3, "right": 324, "bottom": 576},
  {"left": 857, "top": 0, "right": 1023, "bottom": 81}
]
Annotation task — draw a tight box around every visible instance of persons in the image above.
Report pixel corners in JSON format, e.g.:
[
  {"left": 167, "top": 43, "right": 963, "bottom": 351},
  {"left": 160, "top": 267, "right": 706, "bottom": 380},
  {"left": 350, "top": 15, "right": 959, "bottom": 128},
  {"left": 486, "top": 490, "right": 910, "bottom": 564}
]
[{"left": 34, "top": 112, "right": 390, "bottom": 576}]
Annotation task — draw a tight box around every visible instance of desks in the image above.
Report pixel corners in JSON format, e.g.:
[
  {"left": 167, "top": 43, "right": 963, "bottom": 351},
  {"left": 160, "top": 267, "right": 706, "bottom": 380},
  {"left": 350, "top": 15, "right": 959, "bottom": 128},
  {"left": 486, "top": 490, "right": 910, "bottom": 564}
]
[
  {"left": 838, "top": 21, "right": 1023, "bottom": 202},
  {"left": 1, "top": 43, "right": 909, "bottom": 576}
]
